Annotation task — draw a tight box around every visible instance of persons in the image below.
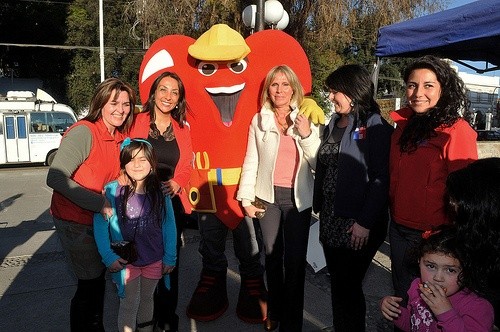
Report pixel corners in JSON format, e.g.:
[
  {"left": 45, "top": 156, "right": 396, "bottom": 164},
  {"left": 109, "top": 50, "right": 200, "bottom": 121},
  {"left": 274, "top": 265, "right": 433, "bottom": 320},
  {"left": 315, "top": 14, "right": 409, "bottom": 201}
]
[
  {"left": 379, "top": 222, "right": 495, "bottom": 332},
  {"left": 92, "top": 137, "right": 178, "bottom": 332},
  {"left": 484, "top": 107, "right": 492, "bottom": 117},
  {"left": 236, "top": 64, "right": 322, "bottom": 332},
  {"left": 470, "top": 107, "right": 478, "bottom": 129},
  {"left": 388, "top": 54, "right": 477, "bottom": 309},
  {"left": 46, "top": 77, "right": 138, "bottom": 332},
  {"left": 118, "top": 71, "right": 195, "bottom": 332},
  {"left": 311, "top": 61, "right": 395, "bottom": 332}
]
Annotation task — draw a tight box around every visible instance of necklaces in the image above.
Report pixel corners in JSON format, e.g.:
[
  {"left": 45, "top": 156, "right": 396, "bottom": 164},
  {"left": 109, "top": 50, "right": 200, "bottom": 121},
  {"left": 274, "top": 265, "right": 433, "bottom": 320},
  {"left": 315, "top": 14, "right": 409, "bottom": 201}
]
[{"left": 272, "top": 106, "right": 292, "bottom": 130}]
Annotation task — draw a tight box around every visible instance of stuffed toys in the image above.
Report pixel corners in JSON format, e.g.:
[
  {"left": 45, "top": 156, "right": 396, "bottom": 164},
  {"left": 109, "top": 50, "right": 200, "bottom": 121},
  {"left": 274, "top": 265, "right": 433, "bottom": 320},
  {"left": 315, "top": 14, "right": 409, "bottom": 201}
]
[{"left": 138, "top": 22, "right": 325, "bottom": 324}]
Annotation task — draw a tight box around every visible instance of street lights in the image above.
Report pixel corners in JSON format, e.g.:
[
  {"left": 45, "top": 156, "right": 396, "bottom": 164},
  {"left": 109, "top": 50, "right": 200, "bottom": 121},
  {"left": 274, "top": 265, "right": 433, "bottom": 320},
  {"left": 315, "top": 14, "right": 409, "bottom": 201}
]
[{"left": 241, "top": 0, "right": 290, "bottom": 32}]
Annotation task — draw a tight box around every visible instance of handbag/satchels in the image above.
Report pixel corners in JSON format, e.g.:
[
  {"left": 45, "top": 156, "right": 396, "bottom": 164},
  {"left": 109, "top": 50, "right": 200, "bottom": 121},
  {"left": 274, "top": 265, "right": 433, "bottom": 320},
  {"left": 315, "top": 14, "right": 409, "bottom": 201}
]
[{"left": 110, "top": 241, "right": 137, "bottom": 265}]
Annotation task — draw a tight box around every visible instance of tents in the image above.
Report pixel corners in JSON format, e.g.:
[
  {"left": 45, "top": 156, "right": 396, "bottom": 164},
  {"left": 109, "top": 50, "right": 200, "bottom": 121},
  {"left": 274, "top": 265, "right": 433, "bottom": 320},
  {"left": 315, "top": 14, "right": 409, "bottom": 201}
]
[{"left": 374, "top": 0, "right": 500, "bottom": 102}]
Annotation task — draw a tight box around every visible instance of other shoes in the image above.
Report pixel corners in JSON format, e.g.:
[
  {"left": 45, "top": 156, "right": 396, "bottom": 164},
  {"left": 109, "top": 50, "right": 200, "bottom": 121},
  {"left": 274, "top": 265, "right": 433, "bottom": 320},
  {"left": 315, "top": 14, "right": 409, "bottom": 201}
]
[{"left": 265, "top": 315, "right": 278, "bottom": 331}]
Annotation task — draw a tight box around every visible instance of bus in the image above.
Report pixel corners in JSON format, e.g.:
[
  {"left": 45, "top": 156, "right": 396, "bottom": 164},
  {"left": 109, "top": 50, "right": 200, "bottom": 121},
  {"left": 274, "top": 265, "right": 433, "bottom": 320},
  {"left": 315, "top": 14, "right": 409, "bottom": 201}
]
[
  {"left": 433, "top": 67, "right": 500, "bottom": 134},
  {"left": 0, "top": 87, "right": 81, "bottom": 170}
]
